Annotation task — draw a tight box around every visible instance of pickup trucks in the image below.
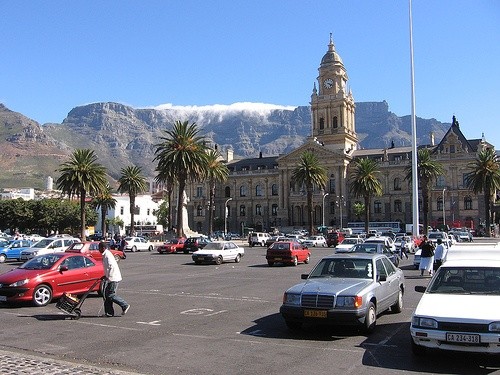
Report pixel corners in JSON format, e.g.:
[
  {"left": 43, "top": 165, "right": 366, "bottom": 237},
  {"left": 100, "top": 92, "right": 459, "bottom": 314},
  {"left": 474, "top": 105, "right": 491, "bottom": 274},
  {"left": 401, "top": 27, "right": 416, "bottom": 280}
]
[
  {"left": 0, "top": 232, "right": 73, "bottom": 240},
  {"left": 212, "top": 231, "right": 240, "bottom": 240},
  {"left": 88, "top": 233, "right": 105, "bottom": 241}
]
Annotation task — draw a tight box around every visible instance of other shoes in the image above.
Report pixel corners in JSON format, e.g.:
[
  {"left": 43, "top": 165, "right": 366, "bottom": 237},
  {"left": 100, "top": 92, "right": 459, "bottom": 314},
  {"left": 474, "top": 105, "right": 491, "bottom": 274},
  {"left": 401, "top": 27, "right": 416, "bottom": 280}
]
[
  {"left": 101, "top": 313, "right": 114, "bottom": 317},
  {"left": 122, "top": 304, "right": 130, "bottom": 315}
]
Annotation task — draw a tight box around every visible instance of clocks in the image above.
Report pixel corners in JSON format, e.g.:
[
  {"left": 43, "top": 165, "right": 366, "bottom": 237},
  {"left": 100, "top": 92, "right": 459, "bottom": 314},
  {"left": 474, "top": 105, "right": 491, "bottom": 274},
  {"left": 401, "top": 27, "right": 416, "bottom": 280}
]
[{"left": 323, "top": 78, "right": 334, "bottom": 89}]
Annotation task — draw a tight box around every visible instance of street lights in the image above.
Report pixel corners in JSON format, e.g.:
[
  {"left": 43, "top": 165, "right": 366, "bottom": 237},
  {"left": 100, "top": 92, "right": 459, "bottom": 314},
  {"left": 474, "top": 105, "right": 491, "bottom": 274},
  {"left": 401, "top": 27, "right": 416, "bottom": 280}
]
[
  {"left": 336, "top": 195, "right": 344, "bottom": 228},
  {"left": 443, "top": 189, "right": 446, "bottom": 225},
  {"left": 322, "top": 192, "right": 329, "bottom": 226},
  {"left": 225, "top": 198, "right": 232, "bottom": 235}
]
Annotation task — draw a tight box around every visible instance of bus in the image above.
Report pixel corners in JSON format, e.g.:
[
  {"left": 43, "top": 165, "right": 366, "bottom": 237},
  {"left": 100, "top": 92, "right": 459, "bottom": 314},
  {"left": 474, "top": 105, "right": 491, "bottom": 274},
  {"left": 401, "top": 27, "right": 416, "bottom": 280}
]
[
  {"left": 125, "top": 225, "right": 164, "bottom": 236},
  {"left": 347, "top": 222, "right": 400, "bottom": 234}
]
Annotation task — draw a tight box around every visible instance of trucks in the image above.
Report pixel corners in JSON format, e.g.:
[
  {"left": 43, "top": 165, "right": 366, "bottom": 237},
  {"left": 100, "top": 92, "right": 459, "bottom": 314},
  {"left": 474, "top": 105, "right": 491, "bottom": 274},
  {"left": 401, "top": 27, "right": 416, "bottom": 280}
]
[{"left": 406, "top": 224, "right": 423, "bottom": 232}]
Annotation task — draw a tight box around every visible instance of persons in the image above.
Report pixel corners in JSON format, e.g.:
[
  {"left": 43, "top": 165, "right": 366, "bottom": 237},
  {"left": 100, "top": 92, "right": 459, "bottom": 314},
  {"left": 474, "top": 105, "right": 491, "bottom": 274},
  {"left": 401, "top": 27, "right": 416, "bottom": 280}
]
[
  {"left": 400, "top": 236, "right": 445, "bottom": 278},
  {"left": 77, "top": 232, "right": 128, "bottom": 253},
  {"left": 98, "top": 243, "right": 131, "bottom": 318}
]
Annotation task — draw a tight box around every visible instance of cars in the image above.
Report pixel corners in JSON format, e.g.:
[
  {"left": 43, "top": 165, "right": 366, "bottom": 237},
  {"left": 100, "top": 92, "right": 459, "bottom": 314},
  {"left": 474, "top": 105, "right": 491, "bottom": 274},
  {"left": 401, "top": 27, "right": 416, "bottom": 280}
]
[
  {"left": 408, "top": 258, "right": 500, "bottom": 355},
  {"left": 65, "top": 241, "right": 126, "bottom": 262},
  {"left": 157, "top": 236, "right": 245, "bottom": 265},
  {"left": 0, "top": 254, "right": 107, "bottom": 306},
  {"left": 280, "top": 254, "right": 405, "bottom": 335},
  {"left": 102, "top": 238, "right": 116, "bottom": 244},
  {"left": 267, "top": 226, "right": 352, "bottom": 248},
  {"left": 335, "top": 227, "right": 475, "bottom": 266},
  {"left": 265, "top": 241, "right": 311, "bottom": 266},
  {"left": 123, "top": 237, "right": 154, "bottom": 252},
  {"left": 478, "top": 224, "right": 495, "bottom": 231},
  {"left": 0, "top": 239, "right": 33, "bottom": 264}
]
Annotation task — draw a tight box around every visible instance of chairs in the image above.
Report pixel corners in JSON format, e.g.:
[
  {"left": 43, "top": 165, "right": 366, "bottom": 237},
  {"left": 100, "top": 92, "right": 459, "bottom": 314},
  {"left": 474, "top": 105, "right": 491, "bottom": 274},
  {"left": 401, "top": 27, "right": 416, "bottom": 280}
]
[
  {"left": 446, "top": 276, "right": 464, "bottom": 288},
  {"left": 333, "top": 262, "right": 347, "bottom": 277},
  {"left": 483, "top": 276, "right": 500, "bottom": 291},
  {"left": 345, "top": 261, "right": 356, "bottom": 276}
]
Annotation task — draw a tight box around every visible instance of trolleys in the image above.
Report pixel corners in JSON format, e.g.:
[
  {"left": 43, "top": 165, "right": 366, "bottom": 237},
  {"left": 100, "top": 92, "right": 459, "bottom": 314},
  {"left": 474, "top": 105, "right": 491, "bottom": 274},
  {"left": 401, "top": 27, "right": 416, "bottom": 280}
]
[{"left": 67, "top": 275, "right": 103, "bottom": 319}]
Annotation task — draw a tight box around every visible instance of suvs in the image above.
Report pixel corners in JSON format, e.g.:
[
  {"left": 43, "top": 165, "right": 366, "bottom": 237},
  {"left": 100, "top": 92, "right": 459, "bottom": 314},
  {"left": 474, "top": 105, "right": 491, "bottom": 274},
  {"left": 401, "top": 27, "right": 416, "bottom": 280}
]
[
  {"left": 249, "top": 232, "right": 272, "bottom": 246},
  {"left": 20, "top": 238, "right": 82, "bottom": 267}
]
